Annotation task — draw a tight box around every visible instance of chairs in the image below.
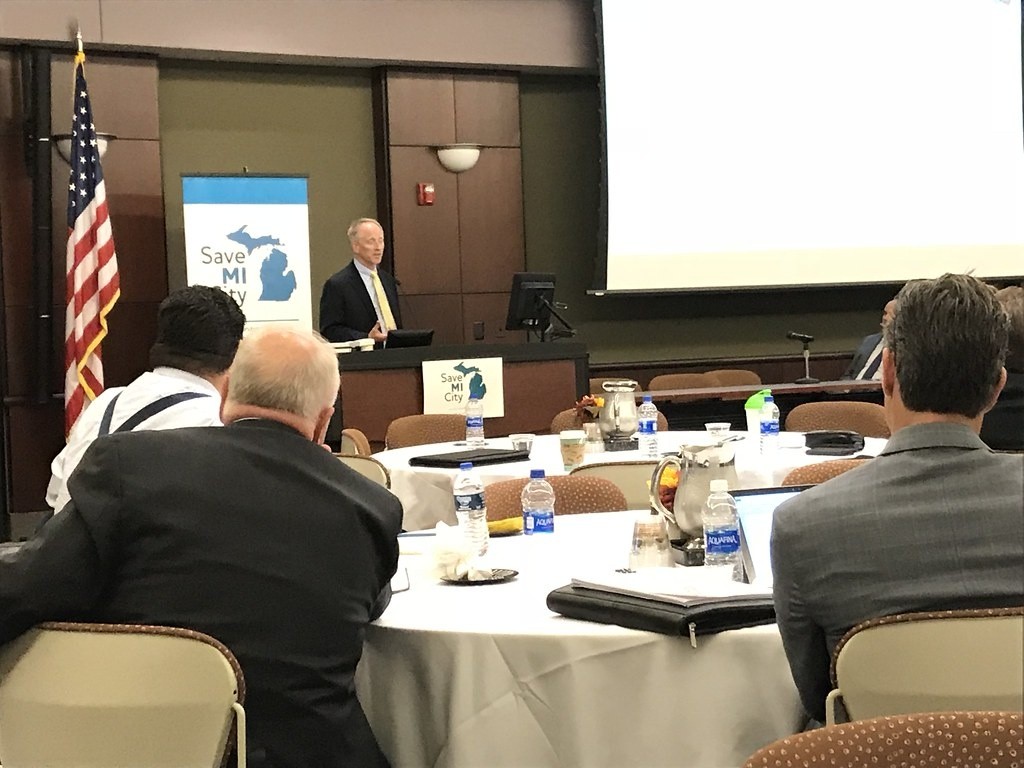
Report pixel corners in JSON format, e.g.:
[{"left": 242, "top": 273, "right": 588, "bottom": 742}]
[{"left": 0, "top": 370, "right": 1024, "bottom": 768}]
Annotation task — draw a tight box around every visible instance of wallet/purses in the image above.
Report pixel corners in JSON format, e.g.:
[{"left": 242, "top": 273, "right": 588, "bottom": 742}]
[{"left": 804, "top": 431, "right": 865, "bottom": 455}]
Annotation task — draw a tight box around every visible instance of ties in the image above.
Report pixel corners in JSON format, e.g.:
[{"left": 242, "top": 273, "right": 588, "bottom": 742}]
[
  {"left": 370, "top": 272, "right": 397, "bottom": 332},
  {"left": 861, "top": 351, "right": 882, "bottom": 380}
]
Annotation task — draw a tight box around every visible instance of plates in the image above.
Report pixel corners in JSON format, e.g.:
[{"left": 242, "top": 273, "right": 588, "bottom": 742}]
[{"left": 440, "top": 568, "right": 520, "bottom": 585}]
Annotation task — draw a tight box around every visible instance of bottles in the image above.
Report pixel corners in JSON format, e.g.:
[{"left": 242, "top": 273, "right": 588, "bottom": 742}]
[
  {"left": 701, "top": 479, "right": 745, "bottom": 583},
  {"left": 636, "top": 395, "right": 658, "bottom": 458},
  {"left": 452, "top": 460, "right": 490, "bottom": 557},
  {"left": 521, "top": 469, "right": 556, "bottom": 573},
  {"left": 465, "top": 394, "right": 485, "bottom": 447},
  {"left": 759, "top": 396, "right": 780, "bottom": 460}
]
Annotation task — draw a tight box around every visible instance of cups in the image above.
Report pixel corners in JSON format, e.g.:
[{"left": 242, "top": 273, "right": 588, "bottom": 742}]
[
  {"left": 560, "top": 430, "right": 586, "bottom": 471},
  {"left": 745, "top": 389, "right": 772, "bottom": 450},
  {"left": 628, "top": 515, "right": 676, "bottom": 571},
  {"left": 509, "top": 432, "right": 536, "bottom": 456},
  {"left": 705, "top": 422, "right": 731, "bottom": 443}
]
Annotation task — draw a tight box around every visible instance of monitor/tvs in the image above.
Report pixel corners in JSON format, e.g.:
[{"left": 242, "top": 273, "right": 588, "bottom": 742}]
[{"left": 505, "top": 270, "right": 556, "bottom": 331}]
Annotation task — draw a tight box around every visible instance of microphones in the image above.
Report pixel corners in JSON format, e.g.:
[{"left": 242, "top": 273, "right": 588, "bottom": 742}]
[{"left": 786, "top": 331, "right": 815, "bottom": 343}]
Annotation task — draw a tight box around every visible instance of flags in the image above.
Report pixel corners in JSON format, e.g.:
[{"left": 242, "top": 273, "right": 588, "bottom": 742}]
[{"left": 65, "top": 53, "right": 119, "bottom": 444}]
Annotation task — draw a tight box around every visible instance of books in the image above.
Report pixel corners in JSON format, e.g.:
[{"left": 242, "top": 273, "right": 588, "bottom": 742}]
[{"left": 569, "top": 563, "right": 775, "bottom": 609}]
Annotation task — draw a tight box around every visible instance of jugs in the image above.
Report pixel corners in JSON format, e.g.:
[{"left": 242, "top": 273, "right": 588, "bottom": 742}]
[
  {"left": 650, "top": 444, "right": 740, "bottom": 539},
  {"left": 596, "top": 392, "right": 637, "bottom": 441}
]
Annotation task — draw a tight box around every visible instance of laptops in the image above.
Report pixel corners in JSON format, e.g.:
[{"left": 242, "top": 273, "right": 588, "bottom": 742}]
[{"left": 384, "top": 329, "right": 435, "bottom": 349}]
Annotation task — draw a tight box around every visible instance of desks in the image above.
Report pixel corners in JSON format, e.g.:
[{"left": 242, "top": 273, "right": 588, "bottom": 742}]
[
  {"left": 368, "top": 430, "right": 888, "bottom": 489},
  {"left": 355, "top": 509, "right": 804, "bottom": 768}
]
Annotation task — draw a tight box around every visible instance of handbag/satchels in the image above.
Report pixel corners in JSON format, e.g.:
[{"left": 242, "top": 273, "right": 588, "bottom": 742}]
[{"left": 547, "top": 584, "right": 776, "bottom": 647}]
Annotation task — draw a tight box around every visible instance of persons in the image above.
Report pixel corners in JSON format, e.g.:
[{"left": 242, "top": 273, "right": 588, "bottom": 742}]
[
  {"left": 2, "top": 324, "right": 403, "bottom": 768},
  {"left": 44, "top": 284, "right": 246, "bottom": 517},
  {"left": 978, "top": 286, "right": 1024, "bottom": 452},
  {"left": 318, "top": 218, "right": 403, "bottom": 350},
  {"left": 828, "top": 298, "right": 896, "bottom": 407},
  {"left": 769, "top": 274, "right": 1024, "bottom": 729}
]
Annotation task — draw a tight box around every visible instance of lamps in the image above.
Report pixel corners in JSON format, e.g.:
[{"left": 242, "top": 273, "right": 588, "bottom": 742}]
[
  {"left": 429, "top": 144, "right": 486, "bottom": 171},
  {"left": 52, "top": 132, "right": 117, "bottom": 163}
]
